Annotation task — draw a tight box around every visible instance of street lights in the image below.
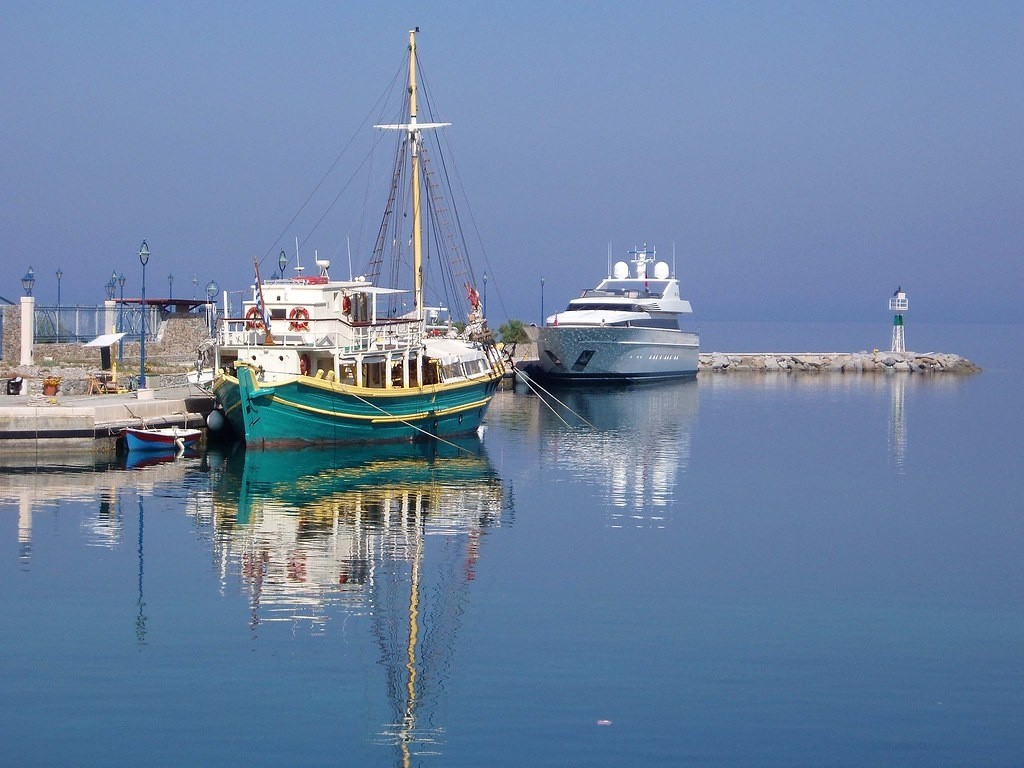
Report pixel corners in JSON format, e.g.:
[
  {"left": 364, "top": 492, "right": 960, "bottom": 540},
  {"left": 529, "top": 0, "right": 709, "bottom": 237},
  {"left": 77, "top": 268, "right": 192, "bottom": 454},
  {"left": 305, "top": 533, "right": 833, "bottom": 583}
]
[
  {"left": 483, "top": 270, "right": 489, "bottom": 318},
  {"left": 56, "top": 268, "right": 63, "bottom": 343},
  {"left": 168, "top": 273, "right": 174, "bottom": 315},
  {"left": 112, "top": 270, "right": 117, "bottom": 300},
  {"left": 205, "top": 280, "right": 220, "bottom": 338},
  {"left": 118, "top": 273, "right": 126, "bottom": 361},
  {"left": 138, "top": 240, "right": 152, "bottom": 390},
  {"left": 28, "top": 265, "right": 33, "bottom": 279},
  {"left": 279, "top": 250, "right": 287, "bottom": 280},
  {"left": 539, "top": 274, "right": 545, "bottom": 326}
]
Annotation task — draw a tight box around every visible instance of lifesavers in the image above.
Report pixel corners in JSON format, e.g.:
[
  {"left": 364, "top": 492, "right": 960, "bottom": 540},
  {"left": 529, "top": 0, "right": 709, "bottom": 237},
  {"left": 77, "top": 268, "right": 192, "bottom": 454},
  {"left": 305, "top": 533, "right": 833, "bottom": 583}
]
[
  {"left": 301, "top": 354, "right": 312, "bottom": 376},
  {"left": 427, "top": 329, "right": 442, "bottom": 339},
  {"left": 289, "top": 307, "right": 309, "bottom": 329},
  {"left": 343, "top": 297, "right": 351, "bottom": 312},
  {"left": 246, "top": 307, "right": 264, "bottom": 327}
]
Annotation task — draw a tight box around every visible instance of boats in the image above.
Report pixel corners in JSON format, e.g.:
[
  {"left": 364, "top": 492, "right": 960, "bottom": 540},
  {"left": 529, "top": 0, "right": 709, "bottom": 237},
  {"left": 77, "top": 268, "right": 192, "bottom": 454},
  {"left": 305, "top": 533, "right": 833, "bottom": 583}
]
[
  {"left": 117, "top": 449, "right": 202, "bottom": 473},
  {"left": 119, "top": 425, "right": 203, "bottom": 452},
  {"left": 207, "top": 440, "right": 514, "bottom": 767},
  {"left": 537, "top": 241, "right": 701, "bottom": 384},
  {"left": 212, "top": 28, "right": 508, "bottom": 453}
]
